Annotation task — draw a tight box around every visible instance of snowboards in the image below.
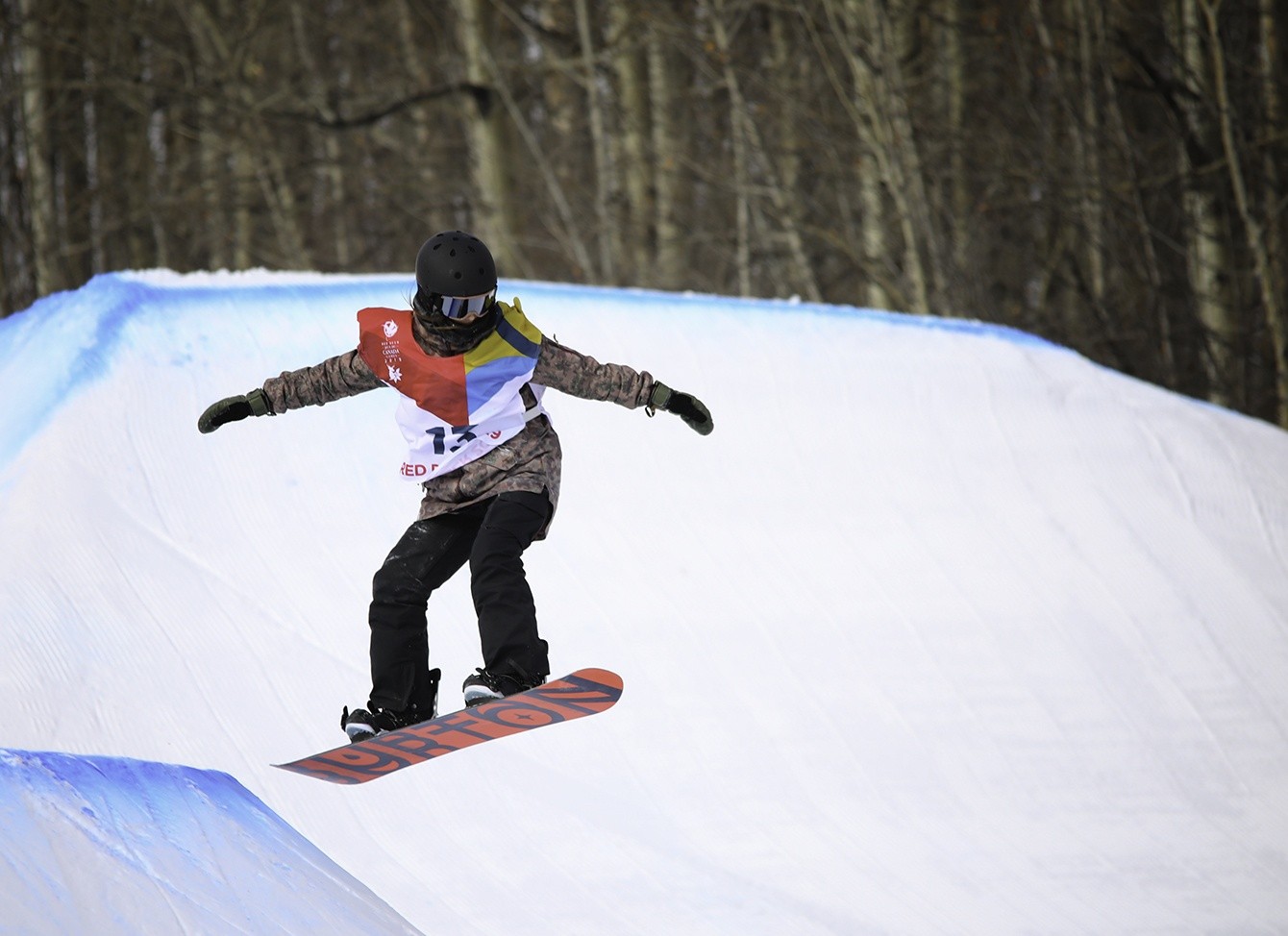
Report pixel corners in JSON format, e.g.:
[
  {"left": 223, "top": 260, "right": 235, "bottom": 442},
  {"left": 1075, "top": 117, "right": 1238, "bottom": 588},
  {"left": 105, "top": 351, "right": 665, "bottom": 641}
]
[{"left": 268, "top": 667, "right": 623, "bottom": 785}]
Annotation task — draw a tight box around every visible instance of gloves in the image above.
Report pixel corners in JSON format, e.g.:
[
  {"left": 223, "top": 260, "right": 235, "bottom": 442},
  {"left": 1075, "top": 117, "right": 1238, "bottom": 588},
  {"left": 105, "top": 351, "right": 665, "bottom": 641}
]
[
  {"left": 198, "top": 388, "right": 269, "bottom": 434},
  {"left": 648, "top": 380, "right": 713, "bottom": 436}
]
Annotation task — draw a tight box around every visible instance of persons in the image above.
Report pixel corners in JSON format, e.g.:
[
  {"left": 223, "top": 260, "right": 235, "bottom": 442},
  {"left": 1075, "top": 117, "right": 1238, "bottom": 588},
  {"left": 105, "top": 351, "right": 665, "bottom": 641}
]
[{"left": 196, "top": 231, "right": 715, "bottom": 742}]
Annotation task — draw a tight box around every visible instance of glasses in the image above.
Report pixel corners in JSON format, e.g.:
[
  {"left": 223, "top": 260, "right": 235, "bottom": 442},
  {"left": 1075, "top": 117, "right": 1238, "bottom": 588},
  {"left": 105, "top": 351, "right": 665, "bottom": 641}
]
[{"left": 430, "top": 285, "right": 496, "bottom": 321}]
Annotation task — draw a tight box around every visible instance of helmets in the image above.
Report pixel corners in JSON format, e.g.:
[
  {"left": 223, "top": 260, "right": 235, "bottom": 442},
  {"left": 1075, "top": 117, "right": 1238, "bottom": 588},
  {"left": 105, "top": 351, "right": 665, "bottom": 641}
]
[{"left": 416, "top": 232, "right": 497, "bottom": 310}]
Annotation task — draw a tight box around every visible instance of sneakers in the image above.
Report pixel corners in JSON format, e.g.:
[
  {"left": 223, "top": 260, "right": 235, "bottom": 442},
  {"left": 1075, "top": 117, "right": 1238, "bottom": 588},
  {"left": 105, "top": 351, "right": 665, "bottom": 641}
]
[
  {"left": 463, "top": 640, "right": 547, "bottom": 706},
  {"left": 346, "top": 667, "right": 441, "bottom": 744}
]
